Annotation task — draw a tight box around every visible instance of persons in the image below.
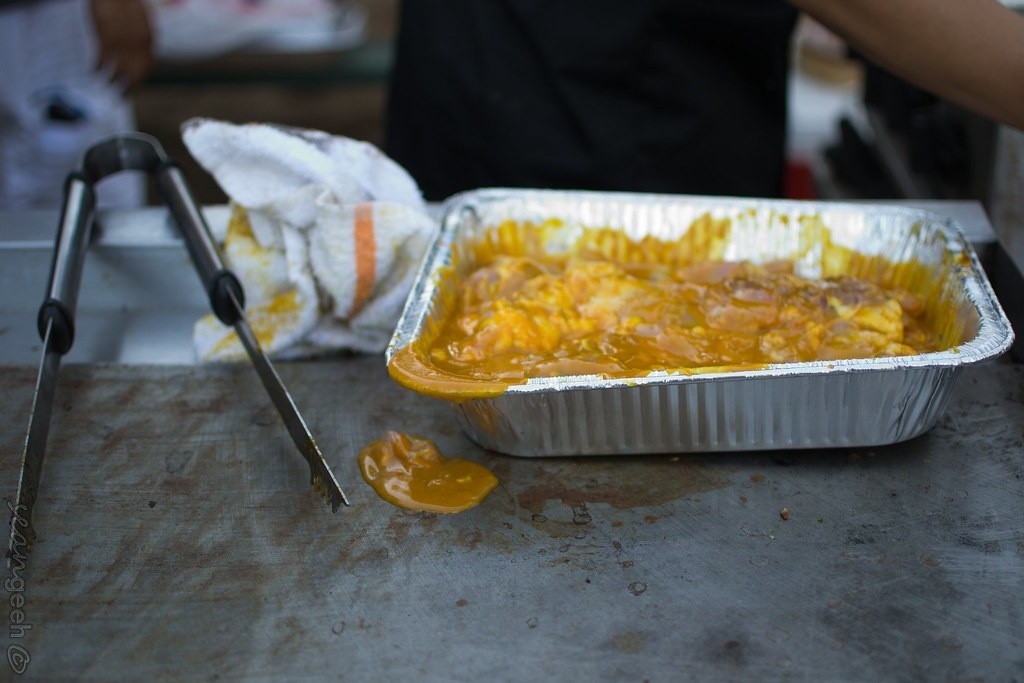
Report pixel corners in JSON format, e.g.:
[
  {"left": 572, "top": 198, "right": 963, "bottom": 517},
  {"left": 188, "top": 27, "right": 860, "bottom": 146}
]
[{"left": 380, "top": 0, "right": 1024, "bottom": 206}]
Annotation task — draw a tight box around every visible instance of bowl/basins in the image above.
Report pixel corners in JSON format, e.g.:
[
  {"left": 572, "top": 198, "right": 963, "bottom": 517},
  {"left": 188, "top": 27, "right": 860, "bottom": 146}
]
[{"left": 385, "top": 184, "right": 1015, "bottom": 458}]
